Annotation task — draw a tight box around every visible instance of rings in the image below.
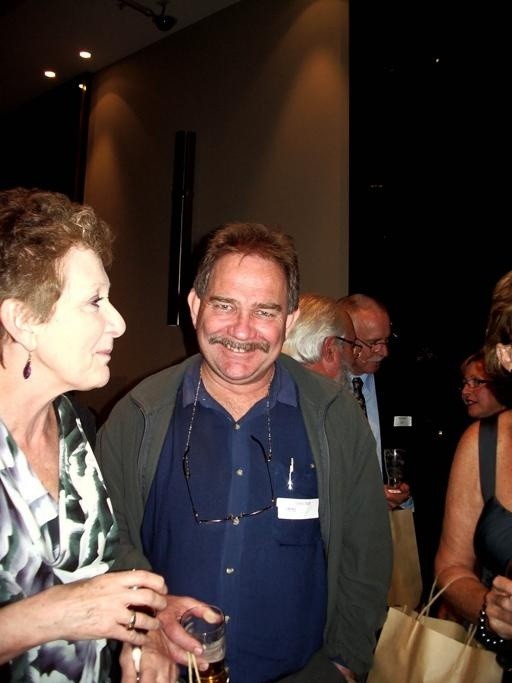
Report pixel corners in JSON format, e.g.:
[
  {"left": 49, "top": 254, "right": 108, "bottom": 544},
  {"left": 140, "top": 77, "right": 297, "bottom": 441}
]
[{"left": 123, "top": 610, "right": 138, "bottom": 633}]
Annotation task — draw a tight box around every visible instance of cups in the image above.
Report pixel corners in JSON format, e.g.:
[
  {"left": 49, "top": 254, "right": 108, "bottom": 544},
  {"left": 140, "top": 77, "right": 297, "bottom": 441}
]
[
  {"left": 180, "top": 605, "right": 229, "bottom": 683},
  {"left": 384, "top": 450, "right": 406, "bottom": 495}
]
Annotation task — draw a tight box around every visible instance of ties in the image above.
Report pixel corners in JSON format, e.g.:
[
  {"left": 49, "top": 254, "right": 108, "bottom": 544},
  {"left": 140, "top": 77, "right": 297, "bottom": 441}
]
[{"left": 352, "top": 378, "right": 368, "bottom": 425}]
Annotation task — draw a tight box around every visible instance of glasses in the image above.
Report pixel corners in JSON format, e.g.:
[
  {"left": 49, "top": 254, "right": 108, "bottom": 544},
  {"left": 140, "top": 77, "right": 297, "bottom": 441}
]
[
  {"left": 181, "top": 435, "right": 277, "bottom": 525},
  {"left": 458, "top": 376, "right": 486, "bottom": 393},
  {"left": 356, "top": 332, "right": 398, "bottom": 353},
  {"left": 333, "top": 335, "right": 362, "bottom": 359}
]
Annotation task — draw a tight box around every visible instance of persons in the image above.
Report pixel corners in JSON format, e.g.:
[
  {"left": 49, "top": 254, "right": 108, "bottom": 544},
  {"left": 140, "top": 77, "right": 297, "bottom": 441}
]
[
  {"left": 333, "top": 290, "right": 424, "bottom": 612},
  {"left": 431, "top": 272, "right": 512, "bottom": 682},
  {"left": 462, "top": 352, "right": 508, "bottom": 419},
  {"left": 281, "top": 295, "right": 361, "bottom": 391},
  {"left": 88, "top": 216, "right": 393, "bottom": 682},
  {"left": 0, "top": 188, "right": 177, "bottom": 681}
]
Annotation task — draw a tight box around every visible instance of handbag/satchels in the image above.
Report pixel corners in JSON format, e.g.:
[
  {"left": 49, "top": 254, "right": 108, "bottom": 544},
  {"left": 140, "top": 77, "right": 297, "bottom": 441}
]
[{"left": 366, "top": 505, "right": 504, "bottom": 683}]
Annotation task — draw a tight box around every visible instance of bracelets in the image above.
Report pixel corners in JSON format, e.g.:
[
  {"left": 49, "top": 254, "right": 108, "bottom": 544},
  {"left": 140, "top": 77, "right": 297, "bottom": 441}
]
[{"left": 477, "top": 600, "right": 506, "bottom": 646}]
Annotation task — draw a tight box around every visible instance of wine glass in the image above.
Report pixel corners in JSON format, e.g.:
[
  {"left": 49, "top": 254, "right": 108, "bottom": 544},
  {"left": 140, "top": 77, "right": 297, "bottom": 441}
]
[{"left": 108, "top": 570, "right": 170, "bottom": 683}]
